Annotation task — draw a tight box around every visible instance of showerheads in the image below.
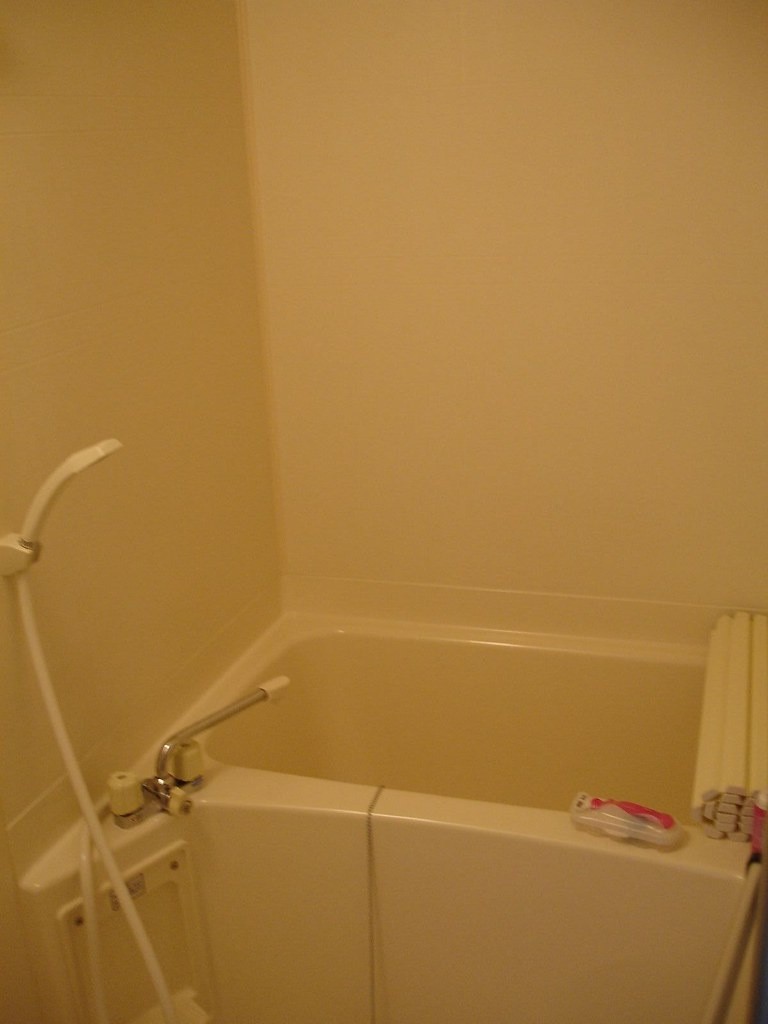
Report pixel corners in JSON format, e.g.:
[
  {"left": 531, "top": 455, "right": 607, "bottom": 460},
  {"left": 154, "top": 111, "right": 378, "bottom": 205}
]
[{"left": 18, "top": 436, "right": 126, "bottom": 551}]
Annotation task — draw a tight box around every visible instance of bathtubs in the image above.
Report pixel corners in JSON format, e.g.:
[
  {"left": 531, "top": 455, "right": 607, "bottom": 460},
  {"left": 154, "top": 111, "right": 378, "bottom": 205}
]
[{"left": 143, "top": 609, "right": 766, "bottom": 1023}]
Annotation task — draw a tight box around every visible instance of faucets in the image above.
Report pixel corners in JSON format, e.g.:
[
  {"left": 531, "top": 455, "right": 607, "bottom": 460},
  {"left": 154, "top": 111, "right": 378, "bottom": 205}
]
[{"left": 156, "top": 674, "right": 292, "bottom": 779}]
